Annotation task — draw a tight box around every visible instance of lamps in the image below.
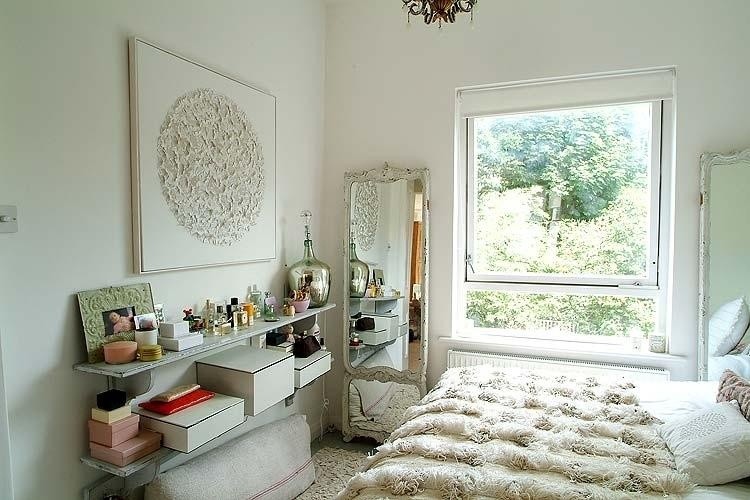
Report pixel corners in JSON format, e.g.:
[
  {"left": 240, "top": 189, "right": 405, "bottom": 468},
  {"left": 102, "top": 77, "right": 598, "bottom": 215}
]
[{"left": 401, "top": 0, "right": 479, "bottom": 31}]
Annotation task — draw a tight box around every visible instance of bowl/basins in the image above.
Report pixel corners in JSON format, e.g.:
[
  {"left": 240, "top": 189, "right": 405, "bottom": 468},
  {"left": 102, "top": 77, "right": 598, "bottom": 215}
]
[{"left": 289, "top": 300, "right": 310, "bottom": 313}]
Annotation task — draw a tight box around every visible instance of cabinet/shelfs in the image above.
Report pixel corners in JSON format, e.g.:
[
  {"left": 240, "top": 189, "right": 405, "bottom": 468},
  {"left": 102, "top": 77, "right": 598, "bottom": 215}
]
[
  {"left": 349, "top": 293, "right": 405, "bottom": 363},
  {"left": 72, "top": 299, "right": 337, "bottom": 473}
]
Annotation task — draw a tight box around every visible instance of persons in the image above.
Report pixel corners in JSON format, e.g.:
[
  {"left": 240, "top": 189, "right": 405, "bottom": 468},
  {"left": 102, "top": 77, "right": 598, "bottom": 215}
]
[{"left": 108, "top": 307, "right": 136, "bottom": 334}]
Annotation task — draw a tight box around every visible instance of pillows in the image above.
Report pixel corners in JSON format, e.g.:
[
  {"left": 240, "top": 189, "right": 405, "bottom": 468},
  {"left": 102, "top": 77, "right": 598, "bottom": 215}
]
[
  {"left": 351, "top": 349, "right": 401, "bottom": 421},
  {"left": 708, "top": 296, "right": 748, "bottom": 356},
  {"left": 143, "top": 412, "right": 316, "bottom": 499},
  {"left": 656, "top": 368, "right": 750, "bottom": 488}
]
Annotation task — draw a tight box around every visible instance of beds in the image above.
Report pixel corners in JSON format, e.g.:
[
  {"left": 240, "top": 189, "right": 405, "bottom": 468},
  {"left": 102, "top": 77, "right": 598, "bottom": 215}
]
[{"left": 344, "top": 365, "right": 748, "bottom": 499}]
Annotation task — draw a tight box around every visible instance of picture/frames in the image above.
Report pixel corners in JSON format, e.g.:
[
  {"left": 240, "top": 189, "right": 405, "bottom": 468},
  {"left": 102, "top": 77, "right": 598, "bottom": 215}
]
[
  {"left": 125, "top": 36, "right": 276, "bottom": 276},
  {"left": 77, "top": 283, "right": 157, "bottom": 365}
]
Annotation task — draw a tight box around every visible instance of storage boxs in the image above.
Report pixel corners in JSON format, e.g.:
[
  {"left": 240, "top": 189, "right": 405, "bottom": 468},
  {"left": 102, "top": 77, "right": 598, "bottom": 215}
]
[
  {"left": 356, "top": 310, "right": 407, "bottom": 348},
  {"left": 82, "top": 343, "right": 333, "bottom": 462}
]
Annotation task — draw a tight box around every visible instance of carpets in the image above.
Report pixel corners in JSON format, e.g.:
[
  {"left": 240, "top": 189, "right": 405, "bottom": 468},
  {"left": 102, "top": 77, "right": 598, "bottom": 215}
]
[
  {"left": 349, "top": 380, "right": 420, "bottom": 434},
  {"left": 294, "top": 444, "right": 369, "bottom": 500}
]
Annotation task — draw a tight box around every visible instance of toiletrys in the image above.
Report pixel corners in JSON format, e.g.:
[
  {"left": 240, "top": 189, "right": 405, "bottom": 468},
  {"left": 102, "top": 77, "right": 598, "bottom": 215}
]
[{"left": 204, "top": 284, "right": 295, "bottom": 336}]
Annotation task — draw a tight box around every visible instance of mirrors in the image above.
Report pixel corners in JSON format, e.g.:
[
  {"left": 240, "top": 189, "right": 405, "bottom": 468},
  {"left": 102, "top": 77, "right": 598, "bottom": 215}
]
[
  {"left": 337, "top": 163, "right": 430, "bottom": 444},
  {"left": 700, "top": 146, "right": 749, "bottom": 380}
]
